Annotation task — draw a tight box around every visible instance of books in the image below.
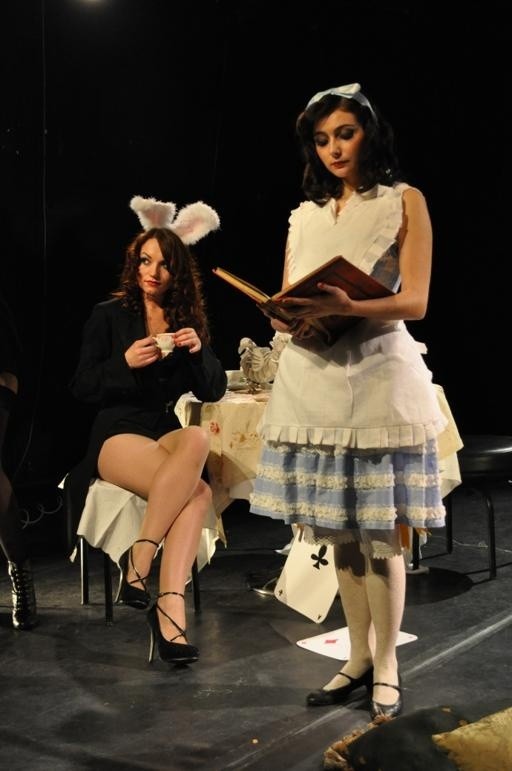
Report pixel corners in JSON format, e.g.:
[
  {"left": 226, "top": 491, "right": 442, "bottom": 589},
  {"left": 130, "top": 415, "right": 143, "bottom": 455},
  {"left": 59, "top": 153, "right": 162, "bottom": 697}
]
[{"left": 210, "top": 254, "right": 398, "bottom": 352}]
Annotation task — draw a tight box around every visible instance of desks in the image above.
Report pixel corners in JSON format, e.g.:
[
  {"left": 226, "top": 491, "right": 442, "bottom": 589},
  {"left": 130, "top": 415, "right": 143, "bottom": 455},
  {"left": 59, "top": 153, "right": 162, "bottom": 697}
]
[{"left": 181, "top": 371, "right": 448, "bottom": 570}]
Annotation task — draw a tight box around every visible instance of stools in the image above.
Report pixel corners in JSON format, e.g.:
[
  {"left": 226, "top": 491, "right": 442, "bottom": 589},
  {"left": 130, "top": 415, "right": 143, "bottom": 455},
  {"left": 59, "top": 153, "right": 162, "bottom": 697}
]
[
  {"left": 444, "top": 434, "right": 512, "bottom": 578},
  {"left": 78, "top": 477, "right": 203, "bottom": 627}
]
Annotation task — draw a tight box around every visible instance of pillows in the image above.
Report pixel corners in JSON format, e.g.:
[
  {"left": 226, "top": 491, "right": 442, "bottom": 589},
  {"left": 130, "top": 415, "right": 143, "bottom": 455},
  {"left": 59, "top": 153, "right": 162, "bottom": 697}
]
[{"left": 322, "top": 702, "right": 512, "bottom": 770}]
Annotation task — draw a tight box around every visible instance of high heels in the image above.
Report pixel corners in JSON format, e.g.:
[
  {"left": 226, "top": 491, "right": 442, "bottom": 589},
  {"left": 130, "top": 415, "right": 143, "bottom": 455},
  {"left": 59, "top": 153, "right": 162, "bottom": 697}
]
[
  {"left": 114, "top": 539, "right": 159, "bottom": 609},
  {"left": 307, "top": 664, "right": 373, "bottom": 706},
  {"left": 371, "top": 670, "right": 403, "bottom": 723},
  {"left": 146, "top": 592, "right": 200, "bottom": 665}
]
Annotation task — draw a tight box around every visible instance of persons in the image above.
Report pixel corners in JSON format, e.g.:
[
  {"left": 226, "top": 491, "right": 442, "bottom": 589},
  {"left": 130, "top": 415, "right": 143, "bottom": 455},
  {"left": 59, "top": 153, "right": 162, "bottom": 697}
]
[
  {"left": 2, "top": 363, "right": 61, "bottom": 630},
  {"left": 62, "top": 226, "right": 230, "bottom": 665},
  {"left": 253, "top": 81, "right": 444, "bottom": 721}
]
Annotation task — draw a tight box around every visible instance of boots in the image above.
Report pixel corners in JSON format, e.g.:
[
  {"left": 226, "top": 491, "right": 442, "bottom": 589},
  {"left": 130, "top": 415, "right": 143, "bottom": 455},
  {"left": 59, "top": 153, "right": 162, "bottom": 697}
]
[{"left": 8, "top": 557, "right": 38, "bottom": 629}]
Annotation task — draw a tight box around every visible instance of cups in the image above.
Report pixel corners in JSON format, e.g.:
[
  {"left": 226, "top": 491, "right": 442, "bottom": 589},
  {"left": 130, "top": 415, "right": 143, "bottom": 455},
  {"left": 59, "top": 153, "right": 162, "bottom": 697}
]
[
  {"left": 224, "top": 369, "right": 274, "bottom": 389},
  {"left": 151, "top": 333, "right": 176, "bottom": 353}
]
